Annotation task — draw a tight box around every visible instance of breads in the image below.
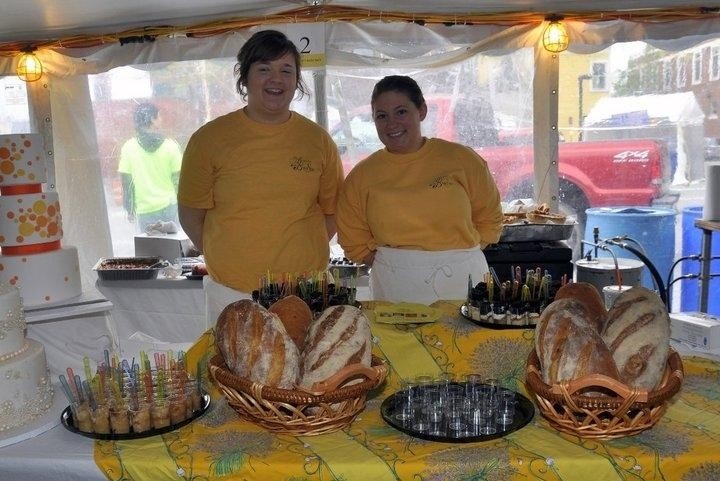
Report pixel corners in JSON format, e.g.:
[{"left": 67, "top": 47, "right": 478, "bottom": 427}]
[
  {"left": 268, "top": 294, "right": 313, "bottom": 356},
  {"left": 553, "top": 281, "right": 607, "bottom": 335},
  {"left": 301, "top": 303, "right": 373, "bottom": 418},
  {"left": 601, "top": 285, "right": 671, "bottom": 392},
  {"left": 215, "top": 299, "right": 302, "bottom": 391},
  {"left": 535, "top": 297, "right": 619, "bottom": 395}
]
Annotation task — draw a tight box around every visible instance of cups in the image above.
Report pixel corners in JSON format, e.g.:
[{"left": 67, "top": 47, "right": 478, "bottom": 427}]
[
  {"left": 71, "top": 367, "right": 204, "bottom": 435},
  {"left": 396, "top": 375, "right": 520, "bottom": 439}
]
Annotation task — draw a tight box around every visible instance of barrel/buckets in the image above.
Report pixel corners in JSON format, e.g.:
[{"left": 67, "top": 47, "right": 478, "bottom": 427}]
[{"left": 576, "top": 257, "right": 644, "bottom": 301}]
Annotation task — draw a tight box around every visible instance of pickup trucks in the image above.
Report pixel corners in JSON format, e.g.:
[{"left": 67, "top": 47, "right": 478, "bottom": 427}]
[{"left": 328, "top": 96, "right": 680, "bottom": 264}]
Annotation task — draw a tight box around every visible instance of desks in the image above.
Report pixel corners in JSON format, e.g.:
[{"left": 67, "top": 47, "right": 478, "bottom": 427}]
[
  {"left": 694, "top": 219, "right": 719, "bottom": 313},
  {"left": 94, "top": 261, "right": 372, "bottom": 366}
]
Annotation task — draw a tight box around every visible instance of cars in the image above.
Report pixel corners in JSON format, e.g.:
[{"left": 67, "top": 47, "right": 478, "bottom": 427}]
[{"left": 704, "top": 137, "right": 720, "bottom": 160}]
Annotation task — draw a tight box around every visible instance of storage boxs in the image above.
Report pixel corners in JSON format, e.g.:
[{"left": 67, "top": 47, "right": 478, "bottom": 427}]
[{"left": 134, "top": 231, "right": 192, "bottom": 258}]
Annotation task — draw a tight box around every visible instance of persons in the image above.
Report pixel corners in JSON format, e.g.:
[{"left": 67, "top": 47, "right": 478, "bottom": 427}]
[
  {"left": 327, "top": 70, "right": 505, "bottom": 304},
  {"left": 115, "top": 101, "right": 191, "bottom": 234},
  {"left": 173, "top": 26, "right": 345, "bottom": 337}
]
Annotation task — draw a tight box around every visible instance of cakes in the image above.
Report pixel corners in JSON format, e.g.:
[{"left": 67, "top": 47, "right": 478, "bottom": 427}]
[
  {"left": 0, "top": 283, "right": 53, "bottom": 433},
  {"left": 0, "top": 134, "right": 81, "bottom": 309}
]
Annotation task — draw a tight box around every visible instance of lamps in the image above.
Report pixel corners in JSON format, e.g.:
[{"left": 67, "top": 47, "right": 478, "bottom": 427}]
[
  {"left": 15, "top": 47, "right": 42, "bottom": 81},
  {"left": 543, "top": 15, "right": 570, "bottom": 53}
]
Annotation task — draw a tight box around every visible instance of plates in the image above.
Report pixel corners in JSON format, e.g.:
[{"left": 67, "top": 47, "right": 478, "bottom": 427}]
[
  {"left": 59, "top": 387, "right": 213, "bottom": 441},
  {"left": 380, "top": 382, "right": 535, "bottom": 445}
]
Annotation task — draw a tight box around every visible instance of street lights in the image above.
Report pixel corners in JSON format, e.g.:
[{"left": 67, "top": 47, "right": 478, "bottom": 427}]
[{"left": 578, "top": 73, "right": 592, "bottom": 140}]
